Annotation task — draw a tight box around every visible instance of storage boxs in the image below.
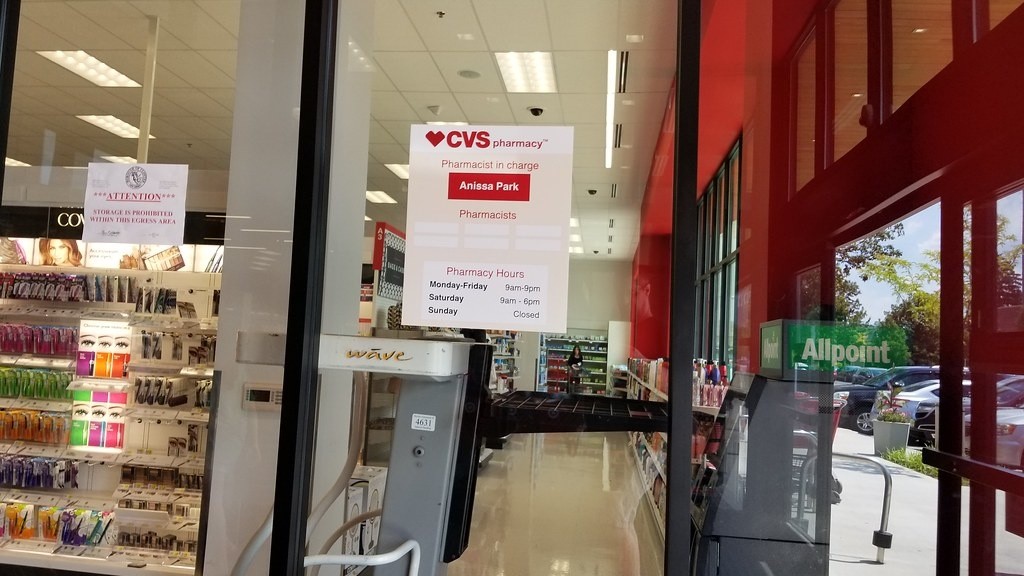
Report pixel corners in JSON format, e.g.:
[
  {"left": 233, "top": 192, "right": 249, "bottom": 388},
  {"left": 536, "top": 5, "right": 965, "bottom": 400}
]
[
  {"left": 1, "top": 272, "right": 220, "bottom": 558},
  {"left": 341, "top": 464, "right": 388, "bottom": 571}
]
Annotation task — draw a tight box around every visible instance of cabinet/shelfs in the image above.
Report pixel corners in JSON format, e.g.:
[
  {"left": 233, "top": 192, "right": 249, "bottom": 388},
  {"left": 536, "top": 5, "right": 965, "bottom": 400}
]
[
  {"left": 0, "top": 262, "right": 221, "bottom": 576},
  {"left": 536, "top": 332, "right": 609, "bottom": 396},
  {"left": 611, "top": 366, "right": 720, "bottom": 544},
  {"left": 363, "top": 327, "right": 523, "bottom": 473}
]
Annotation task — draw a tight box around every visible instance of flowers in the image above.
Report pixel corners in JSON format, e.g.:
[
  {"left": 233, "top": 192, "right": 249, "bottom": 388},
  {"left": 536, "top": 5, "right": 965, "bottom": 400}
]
[{"left": 877, "top": 380, "right": 913, "bottom": 423}]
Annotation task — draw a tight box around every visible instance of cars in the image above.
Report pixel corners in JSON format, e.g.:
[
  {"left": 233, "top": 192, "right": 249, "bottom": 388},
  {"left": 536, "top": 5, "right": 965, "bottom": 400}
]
[
  {"left": 870, "top": 380, "right": 972, "bottom": 430},
  {"left": 962, "top": 405, "right": 1024, "bottom": 470},
  {"left": 833, "top": 368, "right": 972, "bottom": 435},
  {"left": 914, "top": 376, "right": 1024, "bottom": 441},
  {"left": 853, "top": 369, "right": 889, "bottom": 384},
  {"left": 835, "top": 366, "right": 860, "bottom": 381}
]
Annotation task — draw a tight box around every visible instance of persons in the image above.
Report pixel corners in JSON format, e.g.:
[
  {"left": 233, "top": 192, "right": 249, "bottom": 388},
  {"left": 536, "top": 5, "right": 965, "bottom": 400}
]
[
  {"left": 566, "top": 346, "right": 583, "bottom": 394},
  {"left": 39, "top": 238, "right": 82, "bottom": 267}
]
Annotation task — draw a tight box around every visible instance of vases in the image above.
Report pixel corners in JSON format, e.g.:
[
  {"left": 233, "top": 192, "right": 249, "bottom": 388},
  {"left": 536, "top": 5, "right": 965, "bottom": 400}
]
[{"left": 874, "top": 421, "right": 909, "bottom": 457}]
